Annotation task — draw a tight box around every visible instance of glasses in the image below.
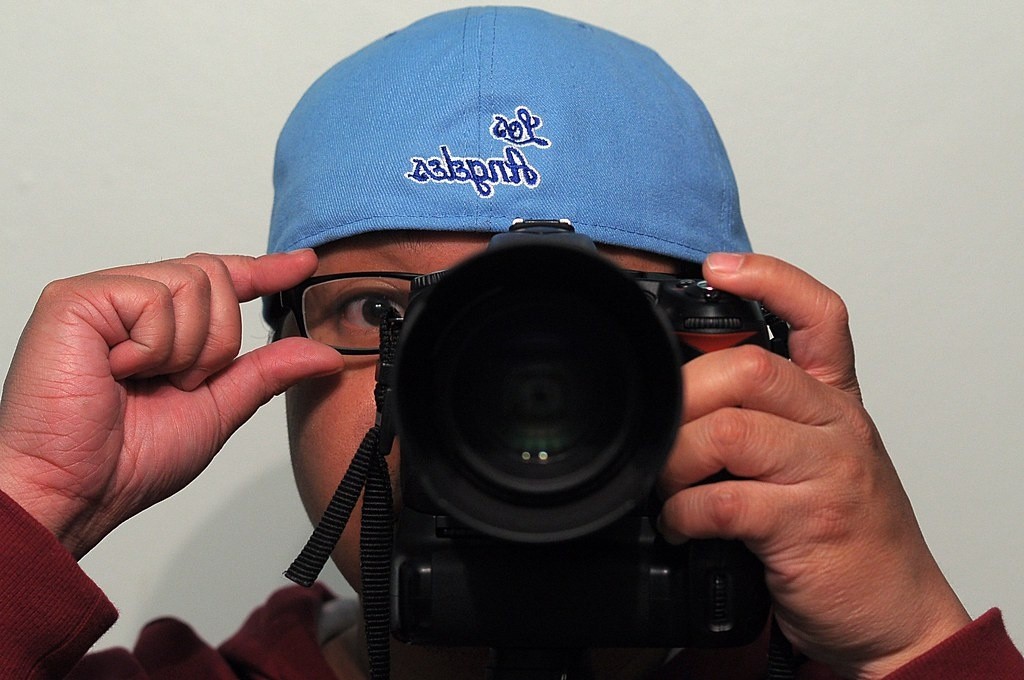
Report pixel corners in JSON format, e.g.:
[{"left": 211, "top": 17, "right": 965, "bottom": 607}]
[{"left": 281, "top": 271, "right": 431, "bottom": 355}]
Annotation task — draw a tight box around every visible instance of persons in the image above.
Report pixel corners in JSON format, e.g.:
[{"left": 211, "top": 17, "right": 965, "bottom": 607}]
[{"left": 0, "top": 6, "right": 1024, "bottom": 679}]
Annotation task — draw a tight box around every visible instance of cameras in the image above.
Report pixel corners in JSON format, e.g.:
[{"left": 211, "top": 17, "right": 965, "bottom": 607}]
[{"left": 374, "top": 219, "right": 771, "bottom": 654}]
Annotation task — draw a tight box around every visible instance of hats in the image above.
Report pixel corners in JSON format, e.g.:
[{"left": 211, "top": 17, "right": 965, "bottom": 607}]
[{"left": 264, "top": 5, "right": 756, "bottom": 263}]
